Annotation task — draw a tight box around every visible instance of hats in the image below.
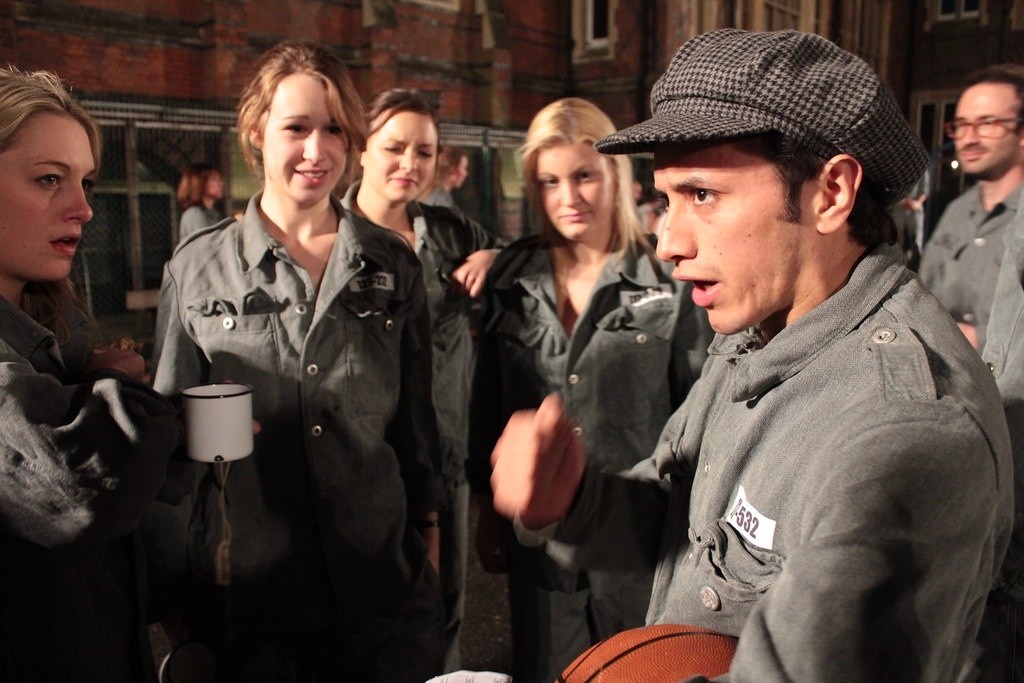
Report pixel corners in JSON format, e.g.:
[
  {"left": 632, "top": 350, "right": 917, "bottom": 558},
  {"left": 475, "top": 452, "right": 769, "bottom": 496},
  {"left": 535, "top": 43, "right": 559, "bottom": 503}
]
[{"left": 593, "top": 27, "right": 931, "bottom": 214}]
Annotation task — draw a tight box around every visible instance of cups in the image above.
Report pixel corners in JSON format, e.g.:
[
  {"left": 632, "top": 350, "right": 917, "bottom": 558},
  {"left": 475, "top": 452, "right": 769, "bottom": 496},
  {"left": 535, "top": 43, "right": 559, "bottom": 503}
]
[{"left": 180, "top": 381, "right": 254, "bottom": 463}]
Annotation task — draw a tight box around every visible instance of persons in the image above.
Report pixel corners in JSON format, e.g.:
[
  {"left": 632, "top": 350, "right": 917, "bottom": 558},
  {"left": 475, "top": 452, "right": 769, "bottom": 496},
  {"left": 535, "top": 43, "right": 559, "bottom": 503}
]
[{"left": 0, "top": 29, "right": 1024, "bottom": 683}]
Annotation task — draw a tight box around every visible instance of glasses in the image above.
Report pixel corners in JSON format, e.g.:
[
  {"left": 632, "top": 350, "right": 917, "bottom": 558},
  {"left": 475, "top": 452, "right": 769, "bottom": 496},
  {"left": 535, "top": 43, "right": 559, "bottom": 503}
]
[{"left": 944, "top": 117, "right": 1023, "bottom": 138}]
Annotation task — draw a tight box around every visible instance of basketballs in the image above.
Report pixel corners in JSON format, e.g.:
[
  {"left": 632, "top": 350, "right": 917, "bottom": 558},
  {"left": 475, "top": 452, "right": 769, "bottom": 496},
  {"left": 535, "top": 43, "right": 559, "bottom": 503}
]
[{"left": 548, "top": 622, "right": 739, "bottom": 683}]
